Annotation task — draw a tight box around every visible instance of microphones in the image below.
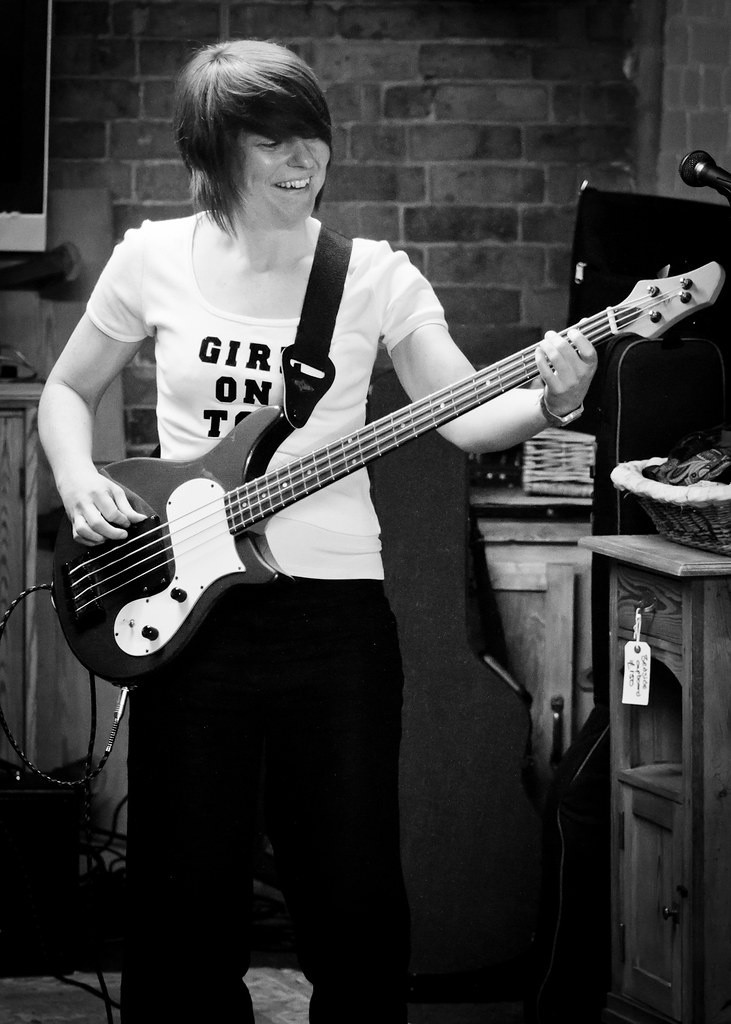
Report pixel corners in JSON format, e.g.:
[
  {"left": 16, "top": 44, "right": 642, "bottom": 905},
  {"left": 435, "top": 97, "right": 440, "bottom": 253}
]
[{"left": 678, "top": 149, "right": 731, "bottom": 209}]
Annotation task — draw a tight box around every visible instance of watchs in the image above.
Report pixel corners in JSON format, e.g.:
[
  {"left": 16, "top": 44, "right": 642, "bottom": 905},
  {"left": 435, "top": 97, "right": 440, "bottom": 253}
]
[{"left": 540, "top": 388, "right": 585, "bottom": 427}]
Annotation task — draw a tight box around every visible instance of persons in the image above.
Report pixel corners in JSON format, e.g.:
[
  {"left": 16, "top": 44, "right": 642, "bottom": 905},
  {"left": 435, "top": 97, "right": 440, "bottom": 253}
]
[{"left": 37, "top": 38, "right": 598, "bottom": 1024}]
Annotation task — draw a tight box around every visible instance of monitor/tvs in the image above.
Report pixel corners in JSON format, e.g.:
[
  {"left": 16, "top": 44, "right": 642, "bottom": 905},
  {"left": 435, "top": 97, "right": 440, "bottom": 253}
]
[{"left": 0, "top": 0, "right": 52, "bottom": 252}]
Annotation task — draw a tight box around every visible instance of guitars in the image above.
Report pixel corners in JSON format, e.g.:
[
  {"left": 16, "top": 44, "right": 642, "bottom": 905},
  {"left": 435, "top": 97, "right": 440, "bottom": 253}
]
[{"left": 47, "top": 253, "right": 727, "bottom": 685}]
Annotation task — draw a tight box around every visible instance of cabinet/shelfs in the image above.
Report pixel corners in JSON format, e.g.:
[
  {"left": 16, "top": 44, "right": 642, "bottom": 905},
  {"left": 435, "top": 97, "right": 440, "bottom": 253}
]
[
  {"left": 0, "top": 378, "right": 45, "bottom": 774},
  {"left": 576, "top": 536, "right": 731, "bottom": 1024}
]
